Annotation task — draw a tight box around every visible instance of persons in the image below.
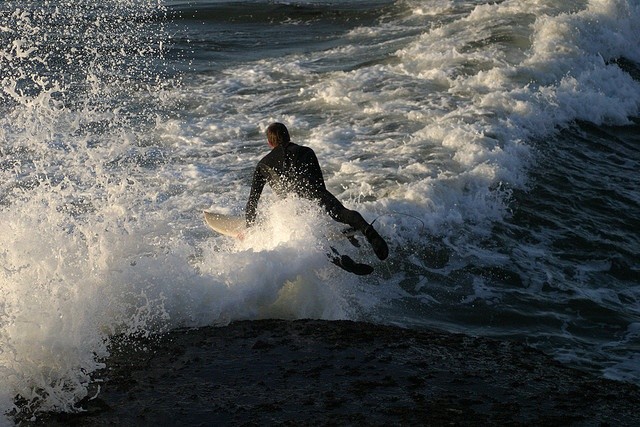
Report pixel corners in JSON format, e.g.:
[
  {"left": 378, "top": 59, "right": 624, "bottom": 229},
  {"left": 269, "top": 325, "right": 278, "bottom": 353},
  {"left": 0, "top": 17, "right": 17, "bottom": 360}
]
[{"left": 245, "top": 121, "right": 389, "bottom": 276}]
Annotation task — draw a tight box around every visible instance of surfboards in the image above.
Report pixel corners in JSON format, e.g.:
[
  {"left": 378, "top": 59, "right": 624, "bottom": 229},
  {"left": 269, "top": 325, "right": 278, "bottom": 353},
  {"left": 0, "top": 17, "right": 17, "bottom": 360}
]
[{"left": 202, "top": 211, "right": 247, "bottom": 237}]
